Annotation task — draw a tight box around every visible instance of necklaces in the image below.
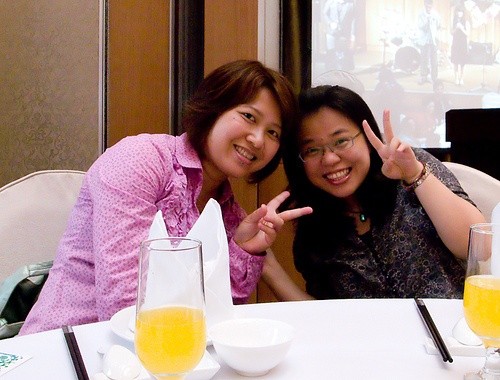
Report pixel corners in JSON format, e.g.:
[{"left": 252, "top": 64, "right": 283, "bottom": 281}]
[{"left": 345, "top": 203, "right": 367, "bottom": 223}]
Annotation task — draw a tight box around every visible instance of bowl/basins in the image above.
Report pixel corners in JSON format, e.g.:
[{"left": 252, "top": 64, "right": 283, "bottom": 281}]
[{"left": 210, "top": 318, "right": 297, "bottom": 377}]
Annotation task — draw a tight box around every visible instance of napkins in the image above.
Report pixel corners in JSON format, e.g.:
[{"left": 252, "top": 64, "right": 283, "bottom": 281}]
[{"left": 148, "top": 198, "right": 234, "bottom": 312}]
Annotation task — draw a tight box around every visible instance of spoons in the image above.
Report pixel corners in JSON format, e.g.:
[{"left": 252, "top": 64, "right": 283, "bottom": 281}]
[
  {"left": 97, "top": 344, "right": 142, "bottom": 380},
  {"left": 454, "top": 316, "right": 483, "bottom": 346}
]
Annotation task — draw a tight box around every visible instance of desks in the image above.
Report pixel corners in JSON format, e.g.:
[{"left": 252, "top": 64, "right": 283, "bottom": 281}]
[{"left": 0, "top": 298, "right": 500, "bottom": 380}]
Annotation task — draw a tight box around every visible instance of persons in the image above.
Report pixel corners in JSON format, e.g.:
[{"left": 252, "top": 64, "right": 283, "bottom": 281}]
[
  {"left": 260, "top": 84, "right": 493, "bottom": 299},
  {"left": 17, "top": 60, "right": 313, "bottom": 336}
]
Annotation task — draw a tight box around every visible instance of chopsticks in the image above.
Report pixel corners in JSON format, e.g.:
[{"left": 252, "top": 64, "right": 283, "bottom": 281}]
[
  {"left": 62, "top": 325, "right": 89, "bottom": 380},
  {"left": 414, "top": 296, "right": 454, "bottom": 363}
]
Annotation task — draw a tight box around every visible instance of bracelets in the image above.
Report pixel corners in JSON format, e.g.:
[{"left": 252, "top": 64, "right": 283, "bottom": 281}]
[{"left": 400, "top": 161, "right": 431, "bottom": 192}]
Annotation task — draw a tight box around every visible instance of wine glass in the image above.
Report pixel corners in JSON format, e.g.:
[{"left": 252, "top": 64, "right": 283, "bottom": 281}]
[{"left": 462, "top": 223, "right": 500, "bottom": 380}]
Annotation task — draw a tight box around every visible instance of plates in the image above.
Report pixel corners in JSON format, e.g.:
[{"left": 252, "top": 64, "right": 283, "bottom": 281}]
[{"left": 110, "top": 305, "right": 213, "bottom": 347}]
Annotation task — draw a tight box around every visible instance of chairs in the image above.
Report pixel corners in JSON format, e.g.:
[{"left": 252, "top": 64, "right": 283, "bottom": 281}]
[{"left": 0, "top": 170, "right": 87, "bottom": 339}]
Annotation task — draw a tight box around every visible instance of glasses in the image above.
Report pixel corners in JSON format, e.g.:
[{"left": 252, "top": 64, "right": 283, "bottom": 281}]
[{"left": 299, "top": 130, "right": 363, "bottom": 162}]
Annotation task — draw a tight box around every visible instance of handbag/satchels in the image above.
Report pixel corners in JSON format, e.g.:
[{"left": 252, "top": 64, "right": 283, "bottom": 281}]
[{"left": 0, "top": 259, "right": 54, "bottom": 342}]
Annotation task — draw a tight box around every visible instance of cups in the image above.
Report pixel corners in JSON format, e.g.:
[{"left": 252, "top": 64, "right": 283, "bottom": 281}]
[{"left": 134, "top": 237, "right": 207, "bottom": 380}]
